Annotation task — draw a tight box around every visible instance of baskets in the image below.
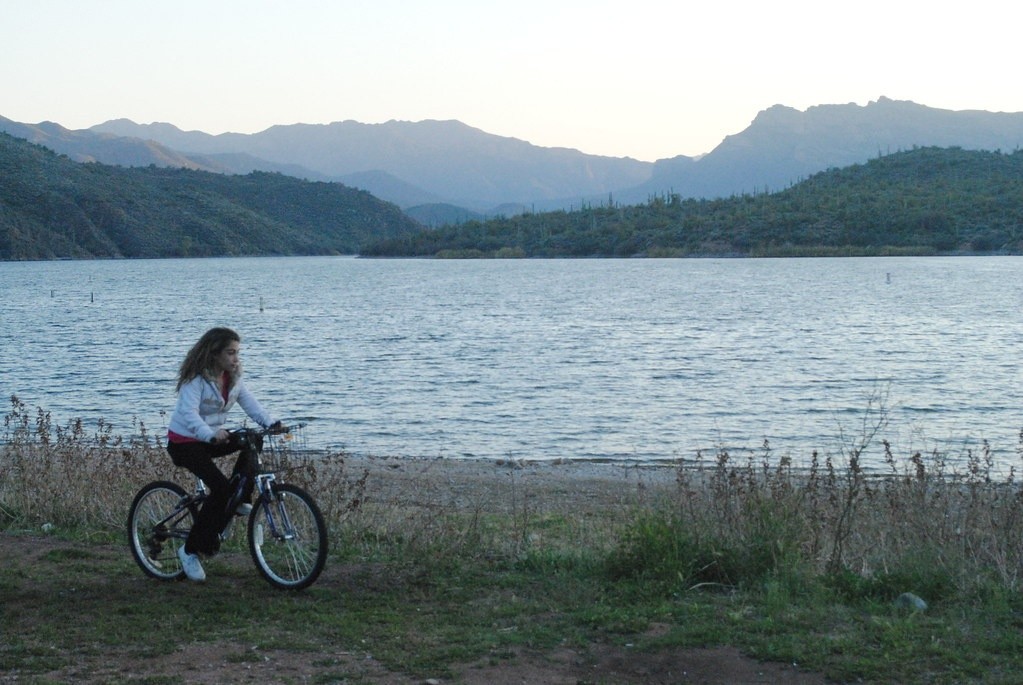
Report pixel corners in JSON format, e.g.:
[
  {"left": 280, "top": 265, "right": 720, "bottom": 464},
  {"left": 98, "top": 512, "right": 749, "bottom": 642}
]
[{"left": 256, "top": 422, "right": 310, "bottom": 473}]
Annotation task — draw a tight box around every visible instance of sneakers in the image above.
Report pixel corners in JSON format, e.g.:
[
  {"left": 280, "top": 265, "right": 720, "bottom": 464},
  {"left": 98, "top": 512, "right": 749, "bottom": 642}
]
[
  {"left": 235, "top": 501, "right": 254, "bottom": 516},
  {"left": 178, "top": 543, "right": 207, "bottom": 582}
]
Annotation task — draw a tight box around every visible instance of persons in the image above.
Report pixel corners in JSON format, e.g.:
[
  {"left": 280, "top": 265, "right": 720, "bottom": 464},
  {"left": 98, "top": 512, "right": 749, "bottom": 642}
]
[{"left": 167, "top": 327, "right": 285, "bottom": 581}]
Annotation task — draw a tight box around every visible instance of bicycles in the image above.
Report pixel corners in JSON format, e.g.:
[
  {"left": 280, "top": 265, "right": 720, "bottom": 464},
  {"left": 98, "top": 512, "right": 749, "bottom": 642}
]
[{"left": 127, "top": 420, "right": 328, "bottom": 594}]
